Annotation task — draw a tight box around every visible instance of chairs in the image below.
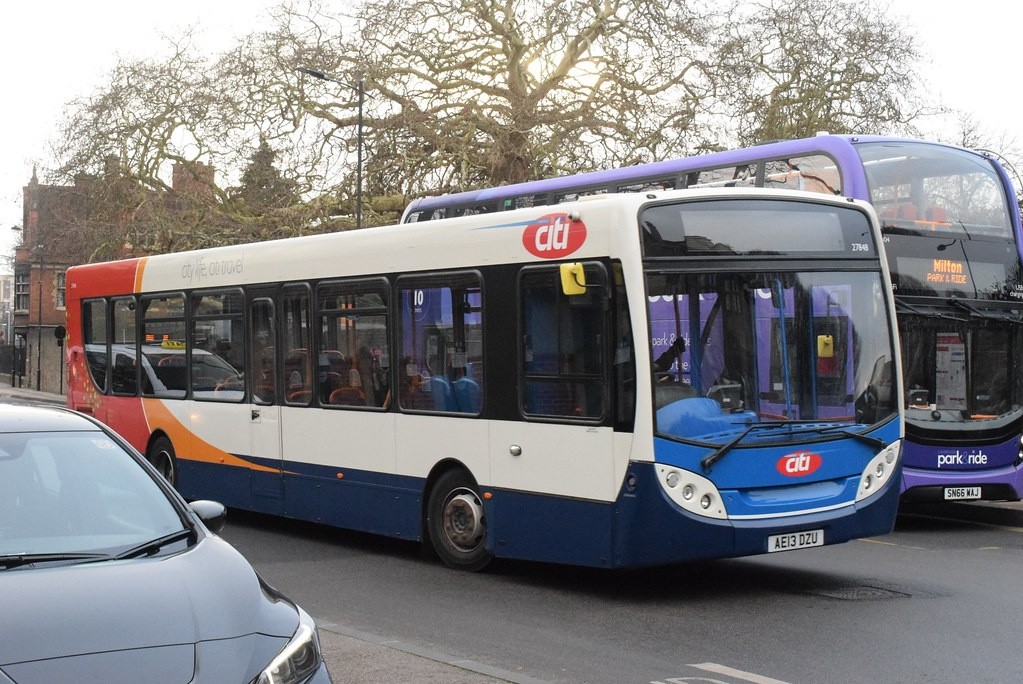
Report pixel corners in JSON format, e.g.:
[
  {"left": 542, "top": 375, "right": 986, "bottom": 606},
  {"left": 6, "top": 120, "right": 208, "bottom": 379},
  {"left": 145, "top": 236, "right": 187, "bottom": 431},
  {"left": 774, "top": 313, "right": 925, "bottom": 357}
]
[
  {"left": 0, "top": 455, "right": 72, "bottom": 539},
  {"left": 156, "top": 347, "right": 484, "bottom": 416}
]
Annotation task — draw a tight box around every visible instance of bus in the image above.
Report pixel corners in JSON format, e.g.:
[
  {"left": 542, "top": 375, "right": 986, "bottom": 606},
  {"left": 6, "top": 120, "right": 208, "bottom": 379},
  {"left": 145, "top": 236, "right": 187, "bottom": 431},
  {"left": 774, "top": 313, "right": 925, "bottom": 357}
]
[
  {"left": 65, "top": 186, "right": 907, "bottom": 575},
  {"left": 397, "top": 134, "right": 1023, "bottom": 522}
]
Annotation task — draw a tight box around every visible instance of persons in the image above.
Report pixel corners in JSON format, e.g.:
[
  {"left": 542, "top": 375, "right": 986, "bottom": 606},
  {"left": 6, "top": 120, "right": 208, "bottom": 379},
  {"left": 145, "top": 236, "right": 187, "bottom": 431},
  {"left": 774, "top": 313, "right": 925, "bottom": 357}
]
[
  {"left": 567, "top": 287, "right": 686, "bottom": 423},
  {"left": 400, "top": 355, "right": 421, "bottom": 409}
]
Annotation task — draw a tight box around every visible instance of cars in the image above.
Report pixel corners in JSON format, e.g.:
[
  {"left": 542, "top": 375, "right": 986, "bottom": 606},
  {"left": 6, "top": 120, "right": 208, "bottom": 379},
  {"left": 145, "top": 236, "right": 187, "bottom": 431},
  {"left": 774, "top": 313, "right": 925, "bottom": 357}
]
[{"left": 0, "top": 395, "right": 333, "bottom": 684}]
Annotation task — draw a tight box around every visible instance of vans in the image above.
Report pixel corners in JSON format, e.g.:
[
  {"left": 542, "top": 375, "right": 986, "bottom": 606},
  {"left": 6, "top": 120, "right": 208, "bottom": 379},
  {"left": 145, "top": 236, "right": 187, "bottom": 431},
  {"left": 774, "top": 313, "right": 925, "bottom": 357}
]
[{"left": 84, "top": 343, "right": 263, "bottom": 405}]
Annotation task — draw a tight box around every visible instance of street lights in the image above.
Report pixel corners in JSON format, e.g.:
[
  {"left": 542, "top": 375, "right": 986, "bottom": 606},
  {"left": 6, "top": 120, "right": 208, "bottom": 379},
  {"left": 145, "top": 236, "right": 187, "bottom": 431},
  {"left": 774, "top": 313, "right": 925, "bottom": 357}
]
[
  {"left": 11, "top": 226, "right": 43, "bottom": 391},
  {"left": 294, "top": 67, "right": 376, "bottom": 230}
]
